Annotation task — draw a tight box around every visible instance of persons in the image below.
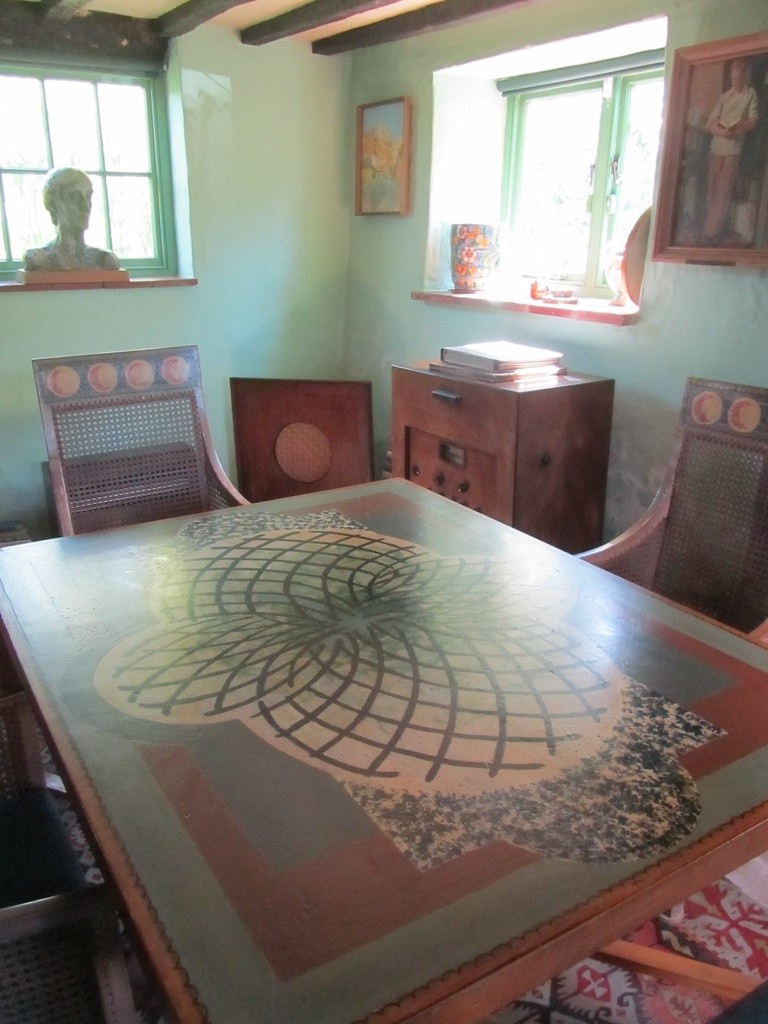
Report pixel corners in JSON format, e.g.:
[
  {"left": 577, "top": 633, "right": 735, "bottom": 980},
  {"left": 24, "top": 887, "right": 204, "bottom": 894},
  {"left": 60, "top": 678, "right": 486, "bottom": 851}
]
[
  {"left": 700, "top": 61, "right": 760, "bottom": 247},
  {"left": 22, "top": 167, "right": 119, "bottom": 271}
]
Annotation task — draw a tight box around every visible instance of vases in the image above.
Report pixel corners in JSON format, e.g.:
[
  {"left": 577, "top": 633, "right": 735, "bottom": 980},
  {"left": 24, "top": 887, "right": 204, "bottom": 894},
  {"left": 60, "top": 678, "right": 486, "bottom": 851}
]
[{"left": 449, "top": 224, "right": 503, "bottom": 291}]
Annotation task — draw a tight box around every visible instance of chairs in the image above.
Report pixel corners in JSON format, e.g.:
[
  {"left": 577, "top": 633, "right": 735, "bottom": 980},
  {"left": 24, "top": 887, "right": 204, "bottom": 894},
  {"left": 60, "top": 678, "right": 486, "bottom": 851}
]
[
  {"left": 31, "top": 345, "right": 251, "bottom": 537},
  {"left": 575, "top": 376, "right": 768, "bottom": 645}
]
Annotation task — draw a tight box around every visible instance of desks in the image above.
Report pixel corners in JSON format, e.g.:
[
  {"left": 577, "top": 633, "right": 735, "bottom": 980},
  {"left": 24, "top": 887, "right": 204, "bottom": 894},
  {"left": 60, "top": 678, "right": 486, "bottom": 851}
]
[{"left": 0, "top": 476, "right": 768, "bottom": 1024}]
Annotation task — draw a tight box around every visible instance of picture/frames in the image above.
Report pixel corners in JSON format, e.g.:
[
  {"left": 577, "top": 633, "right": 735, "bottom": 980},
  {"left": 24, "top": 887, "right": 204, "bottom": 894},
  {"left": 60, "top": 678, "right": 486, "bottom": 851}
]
[
  {"left": 652, "top": 30, "right": 768, "bottom": 268},
  {"left": 355, "top": 94, "right": 413, "bottom": 216}
]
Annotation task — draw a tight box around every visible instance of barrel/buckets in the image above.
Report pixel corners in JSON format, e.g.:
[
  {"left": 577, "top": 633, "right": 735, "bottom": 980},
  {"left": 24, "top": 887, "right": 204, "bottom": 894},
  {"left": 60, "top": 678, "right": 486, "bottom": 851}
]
[{"left": 452, "top": 223, "right": 499, "bottom": 292}]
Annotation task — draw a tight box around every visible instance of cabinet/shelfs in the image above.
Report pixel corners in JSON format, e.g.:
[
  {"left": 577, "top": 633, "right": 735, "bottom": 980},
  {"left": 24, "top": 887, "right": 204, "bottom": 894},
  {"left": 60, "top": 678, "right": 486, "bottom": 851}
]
[{"left": 390, "top": 359, "right": 616, "bottom": 554}]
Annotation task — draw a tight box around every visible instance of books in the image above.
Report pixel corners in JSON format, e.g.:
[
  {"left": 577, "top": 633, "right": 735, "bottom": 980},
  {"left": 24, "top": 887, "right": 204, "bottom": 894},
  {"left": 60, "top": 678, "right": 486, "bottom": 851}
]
[
  {"left": 717, "top": 118, "right": 742, "bottom": 130},
  {"left": 429, "top": 341, "right": 566, "bottom": 384}
]
